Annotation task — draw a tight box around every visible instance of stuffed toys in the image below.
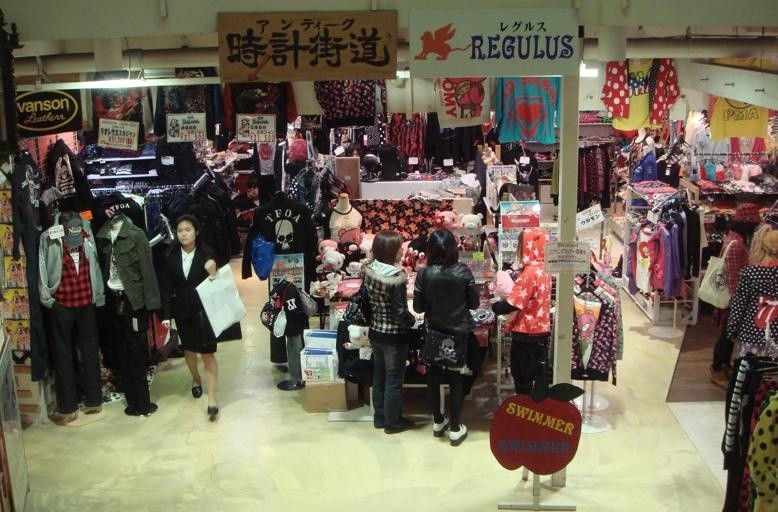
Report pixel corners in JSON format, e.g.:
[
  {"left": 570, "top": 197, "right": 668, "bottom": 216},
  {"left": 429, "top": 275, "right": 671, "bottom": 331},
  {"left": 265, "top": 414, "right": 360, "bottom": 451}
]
[
  {"left": 315, "top": 236, "right": 428, "bottom": 278},
  {"left": 456, "top": 213, "right": 485, "bottom": 233},
  {"left": 435, "top": 209, "right": 459, "bottom": 231},
  {"left": 494, "top": 270, "right": 519, "bottom": 322}
]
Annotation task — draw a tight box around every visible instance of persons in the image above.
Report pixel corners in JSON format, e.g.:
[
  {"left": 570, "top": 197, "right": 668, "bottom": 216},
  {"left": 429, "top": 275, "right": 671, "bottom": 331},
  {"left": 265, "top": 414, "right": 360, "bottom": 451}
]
[
  {"left": 375, "top": 143, "right": 404, "bottom": 181},
  {"left": 344, "top": 142, "right": 362, "bottom": 158},
  {"left": 240, "top": 189, "right": 318, "bottom": 296},
  {"left": 707, "top": 202, "right": 761, "bottom": 391},
  {"left": 411, "top": 228, "right": 480, "bottom": 447},
  {"left": 630, "top": 145, "right": 659, "bottom": 207},
  {"left": 94, "top": 210, "right": 162, "bottom": 417},
  {"left": 490, "top": 226, "right": 552, "bottom": 396},
  {"left": 359, "top": 229, "right": 416, "bottom": 435},
  {"left": 724, "top": 224, "right": 777, "bottom": 359},
  {"left": 157, "top": 214, "right": 223, "bottom": 422},
  {"left": 274, "top": 277, "right": 309, "bottom": 392},
  {"left": 327, "top": 191, "right": 363, "bottom": 241},
  {"left": 628, "top": 127, "right": 657, "bottom": 183},
  {"left": 36, "top": 210, "right": 106, "bottom": 425}
]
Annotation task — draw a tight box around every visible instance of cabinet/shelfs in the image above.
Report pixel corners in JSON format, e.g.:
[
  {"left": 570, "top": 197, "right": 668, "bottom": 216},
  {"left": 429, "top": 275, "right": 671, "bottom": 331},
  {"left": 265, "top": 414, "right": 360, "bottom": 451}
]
[
  {"left": 481, "top": 163, "right": 542, "bottom": 396},
  {"left": 82, "top": 154, "right": 164, "bottom": 184},
  {"left": 620, "top": 179, "right": 702, "bottom": 329},
  {"left": 678, "top": 176, "right": 777, "bottom": 318},
  {"left": 523, "top": 136, "right": 560, "bottom": 183}
]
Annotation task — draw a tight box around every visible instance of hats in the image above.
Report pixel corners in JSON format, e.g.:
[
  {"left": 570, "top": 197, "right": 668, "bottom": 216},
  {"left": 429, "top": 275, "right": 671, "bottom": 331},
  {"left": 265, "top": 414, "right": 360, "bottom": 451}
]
[{"left": 730, "top": 202, "right": 767, "bottom": 224}]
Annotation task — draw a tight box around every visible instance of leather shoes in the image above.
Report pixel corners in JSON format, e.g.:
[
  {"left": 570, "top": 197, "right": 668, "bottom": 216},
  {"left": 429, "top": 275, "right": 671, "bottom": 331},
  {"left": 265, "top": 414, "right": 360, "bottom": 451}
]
[
  {"left": 207, "top": 405, "right": 219, "bottom": 415},
  {"left": 192, "top": 384, "right": 202, "bottom": 398},
  {"left": 432, "top": 414, "right": 450, "bottom": 438},
  {"left": 449, "top": 423, "right": 467, "bottom": 447}
]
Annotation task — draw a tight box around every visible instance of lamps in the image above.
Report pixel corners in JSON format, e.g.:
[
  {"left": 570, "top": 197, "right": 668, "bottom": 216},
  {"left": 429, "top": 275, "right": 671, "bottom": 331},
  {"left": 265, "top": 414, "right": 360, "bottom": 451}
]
[{"left": 10, "top": 52, "right": 223, "bottom": 96}]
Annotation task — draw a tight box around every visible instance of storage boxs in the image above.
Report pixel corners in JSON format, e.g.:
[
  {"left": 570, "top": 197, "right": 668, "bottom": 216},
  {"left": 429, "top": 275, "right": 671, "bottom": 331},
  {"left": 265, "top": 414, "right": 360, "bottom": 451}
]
[
  {"left": 537, "top": 183, "right": 560, "bottom": 225},
  {"left": 303, "top": 375, "right": 352, "bottom": 416}
]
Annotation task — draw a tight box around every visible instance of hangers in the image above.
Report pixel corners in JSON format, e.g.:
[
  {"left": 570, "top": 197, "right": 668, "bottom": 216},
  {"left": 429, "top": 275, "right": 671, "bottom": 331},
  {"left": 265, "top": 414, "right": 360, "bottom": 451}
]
[
  {"left": 682, "top": 150, "right": 772, "bottom": 173},
  {"left": 573, "top": 260, "right": 604, "bottom": 304},
  {"left": 748, "top": 334, "right": 777, "bottom": 397},
  {"left": 88, "top": 179, "right": 199, "bottom": 208}
]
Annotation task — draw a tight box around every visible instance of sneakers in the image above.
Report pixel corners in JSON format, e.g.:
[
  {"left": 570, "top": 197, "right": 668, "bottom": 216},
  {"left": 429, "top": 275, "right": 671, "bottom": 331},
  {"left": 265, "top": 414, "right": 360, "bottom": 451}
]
[
  {"left": 707, "top": 363, "right": 734, "bottom": 390},
  {"left": 373, "top": 413, "right": 415, "bottom": 434}
]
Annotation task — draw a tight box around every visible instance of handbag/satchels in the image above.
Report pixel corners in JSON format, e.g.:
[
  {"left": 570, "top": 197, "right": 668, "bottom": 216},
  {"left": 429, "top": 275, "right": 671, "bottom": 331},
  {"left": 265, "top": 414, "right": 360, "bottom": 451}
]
[
  {"left": 260, "top": 301, "right": 287, "bottom": 338},
  {"left": 194, "top": 263, "right": 248, "bottom": 339},
  {"left": 297, "top": 288, "right": 318, "bottom": 318},
  {"left": 249, "top": 231, "right": 277, "bottom": 282},
  {"left": 697, "top": 255, "right": 732, "bottom": 311},
  {"left": 342, "top": 274, "right": 373, "bottom": 327},
  {"left": 420, "top": 328, "right": 470, "bottom": 368}
]
